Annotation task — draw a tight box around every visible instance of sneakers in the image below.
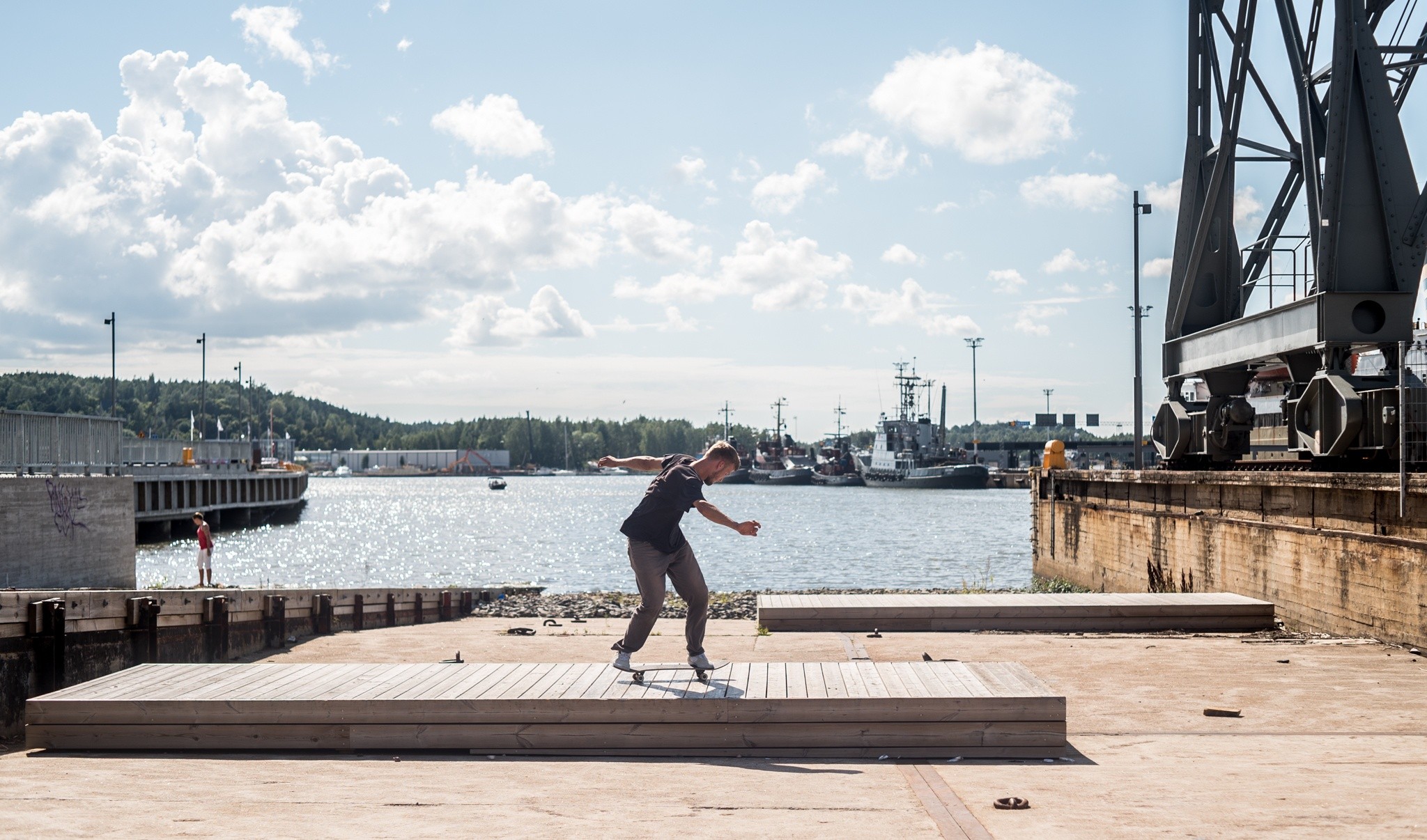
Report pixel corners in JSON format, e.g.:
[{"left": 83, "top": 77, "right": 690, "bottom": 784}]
[
  {"left": 613, "top": 650, "right": 631, "bottom": 671},
  {"left": 688, "top": 653, "right": 714, "bottom": 668}
]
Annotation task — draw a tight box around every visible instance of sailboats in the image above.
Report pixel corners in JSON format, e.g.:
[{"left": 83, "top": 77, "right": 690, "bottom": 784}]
[
  {"left": 849, "top": 355, "right": 989, "bottom": 488},
  {"left": 748, "top": 398, "right": 812, "bottom": 486},
  {"left": 700, "top": 400, "right": 749, "bottom": 485},
  {"left": 809, "top": 397, "right": 862, "bottom": 486}
]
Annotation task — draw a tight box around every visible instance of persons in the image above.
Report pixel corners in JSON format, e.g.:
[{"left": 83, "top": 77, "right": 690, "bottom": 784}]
[
  {"left": 597, "top": 440, "right": 762, "bottom": 671},
  {"left": 192, "top": 512, "right": 216, "bottom": 588}
]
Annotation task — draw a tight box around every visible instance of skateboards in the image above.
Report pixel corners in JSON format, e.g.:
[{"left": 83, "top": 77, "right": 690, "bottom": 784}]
[{"left": 612, "top": 661, "right": 730, "bottom": 682}]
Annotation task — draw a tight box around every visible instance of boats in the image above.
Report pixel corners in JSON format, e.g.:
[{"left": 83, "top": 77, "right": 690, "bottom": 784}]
[
  {"left": 367, "top": 465, "right": 384, "bottom": 476},
  {"left": 261, "top": 457, "right": 279, "bottom": 469},
  {"left": 526, "top": 460, "right": 629, "bottom": 476},
  {"left": 488, "top": 477, "right": 507, "bottom": 490},
  {"left": 308, "top": 464, "right": 353, "bottom": 477}
]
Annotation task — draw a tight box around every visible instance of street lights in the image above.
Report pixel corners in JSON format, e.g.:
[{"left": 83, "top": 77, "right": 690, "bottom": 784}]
[
  {"left": 234, "top": 361, "right": 241, "bottom": 440},
  {"left": 245, "top": 375, "right": 252, "bottom": 441},
  {"left": 1043, "top": 388, "right": 1054, "bottom": 441},
  {"left": 964, "top": 337, "right": 984, "bottom": 464},
  {"left": 196, "top": 333, "right": 205, "bottom": 440},
  {"left": 104, "top": 311, "right": 115, "bottom": 417},
  {"left": 1128, "top": 190, "right": 1151, "bottom": 469}
]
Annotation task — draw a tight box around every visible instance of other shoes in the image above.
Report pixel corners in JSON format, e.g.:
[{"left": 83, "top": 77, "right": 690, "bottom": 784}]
[
  {"left": 194, "top": 584, "right": 204, "bottom": 587},
  {"left": 208, "top": 583, "right": 211, "bottom": 587}
]
